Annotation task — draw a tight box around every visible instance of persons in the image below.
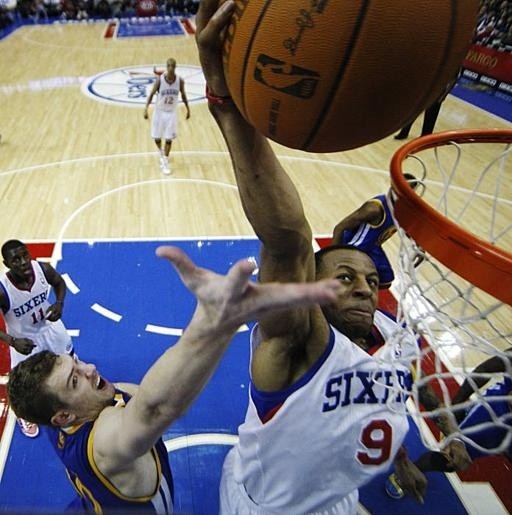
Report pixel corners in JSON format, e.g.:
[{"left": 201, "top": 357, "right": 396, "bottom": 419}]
[
  {"left": 0, "top": 240, "right": 74, "bottom": 438},
  {"left": 195, "top": 0, "right": 472, "bottom": 515},
  {"left": 383, "top": 348, "right": 512, "bottom": 503},
  {"left": 332, "top": 174, "right": 425, "bottom": 288},
  {"left": 472, "top": 0, "right": 512, "bottom": 52},
  {"left": 7, "top": 246, "right": 342, "bottom": 515},
  {"left": 394, "top": 67, "right": 458, "bottom": 139},
  {"left": 143, "top": 58, "right": 190, "bottom": 175},
  {"left": 0, "top": 0, "right": 199, "bottom": 30}
]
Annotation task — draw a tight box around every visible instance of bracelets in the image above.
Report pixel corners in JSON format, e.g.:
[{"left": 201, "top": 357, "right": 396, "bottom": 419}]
[
  {"left": 206, "top": 84, "right": 234, "bottom": 104},
  {"left": 56, "top": 299, "right": 64, "bottom": 304},
  {"left": 9, "top": 337, "right": 16, "bottom": 346}
]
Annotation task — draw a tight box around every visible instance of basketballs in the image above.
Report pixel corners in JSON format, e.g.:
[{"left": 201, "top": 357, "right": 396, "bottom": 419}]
[{"left": 216, "top": 0, "right": 480, "bottom": 152}]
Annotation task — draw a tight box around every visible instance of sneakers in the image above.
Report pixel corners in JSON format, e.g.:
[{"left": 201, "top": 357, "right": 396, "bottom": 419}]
[
  {"left": 160, "top": 157, "right": 171, "bottom": 176},
  {"left": 15, "top": 417, "right": 40, "bottom": 437},
  {"left": 395, "top": 134, "right": 408, "bottom": 139},
  {"left": 384, "top": 473, "right": 407, "bottom": 500}
]
